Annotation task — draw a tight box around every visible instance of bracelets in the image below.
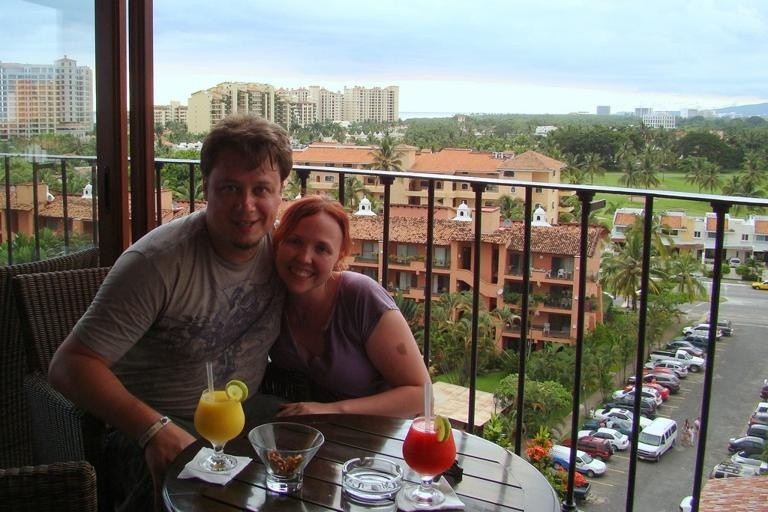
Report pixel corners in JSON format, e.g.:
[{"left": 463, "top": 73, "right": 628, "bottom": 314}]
[{"left": 138, "top": 416, "right": 172, "bottom": 449}]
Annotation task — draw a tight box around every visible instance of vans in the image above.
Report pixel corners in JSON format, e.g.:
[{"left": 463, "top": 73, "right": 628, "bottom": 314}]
[{"left": 728, "top": 256, "right": 741, "bottom": 267}]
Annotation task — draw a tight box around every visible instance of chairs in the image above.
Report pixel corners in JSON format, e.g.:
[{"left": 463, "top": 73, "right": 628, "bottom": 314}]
[
  {"left": 0, "top": 246, "right": 108, "bottom": 468},
  {"left": 0, "top": 461, "right": 99, "bottom": 510},
  {"left": 13, "top": 268, "right": 116, "bottom": 463}
]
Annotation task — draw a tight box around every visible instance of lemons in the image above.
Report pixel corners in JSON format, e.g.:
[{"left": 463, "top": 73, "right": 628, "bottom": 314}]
[
  {"left": 225, "top": 380, "right": 248, "bottom": 402},
  {"left": 434, "top": 415, "right": 451, "bottom": 442}
]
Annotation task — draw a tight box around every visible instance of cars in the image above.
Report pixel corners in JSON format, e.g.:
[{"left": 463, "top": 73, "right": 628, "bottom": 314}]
[
  {"left": 679, "top": 377, "right": 767, "bottom": 512},
  {"left": 670, "top": 272, "right": 704, "bottom": 283},
  {"left": 546, "top": 319, "right": 735, "bottom": 501},
  {"left": 752, "top": 280, "right": 768, "bottom": 290}
]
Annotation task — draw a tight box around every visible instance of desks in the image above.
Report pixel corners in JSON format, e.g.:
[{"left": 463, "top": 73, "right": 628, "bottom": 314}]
[{"left": 159, "top": 416, "right": 563, "bottom": 511}]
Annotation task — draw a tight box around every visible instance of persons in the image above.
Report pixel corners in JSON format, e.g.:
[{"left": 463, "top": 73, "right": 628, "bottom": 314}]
[
  {"left": 268, "top": 194, "right": 433, "bottom": 417},
  {"left": 46, "top": 114, "right": 292, "bottom": 510},
  {"left": 590, "top": 406, "right": 594, "bottom": 417},
  {"left": 598, "top": 419, "right": 605, "bottom": 426},
  {"left": 681, "top": 416, "right": 701, "bottom": 445},
  {"left": 606, "top": 418, "right": 614, "bottom": 428}
]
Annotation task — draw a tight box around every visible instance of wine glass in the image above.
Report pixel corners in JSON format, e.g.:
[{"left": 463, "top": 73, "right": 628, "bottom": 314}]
[
  {"left": 402, "top": 415, "right": 457, "bottom": 507},
  {"left": 195, "top": 388, "right": 246, "bottom": 470},
  {"left": 246, "top": 422, "right": 326, "bottom": 493}
]
